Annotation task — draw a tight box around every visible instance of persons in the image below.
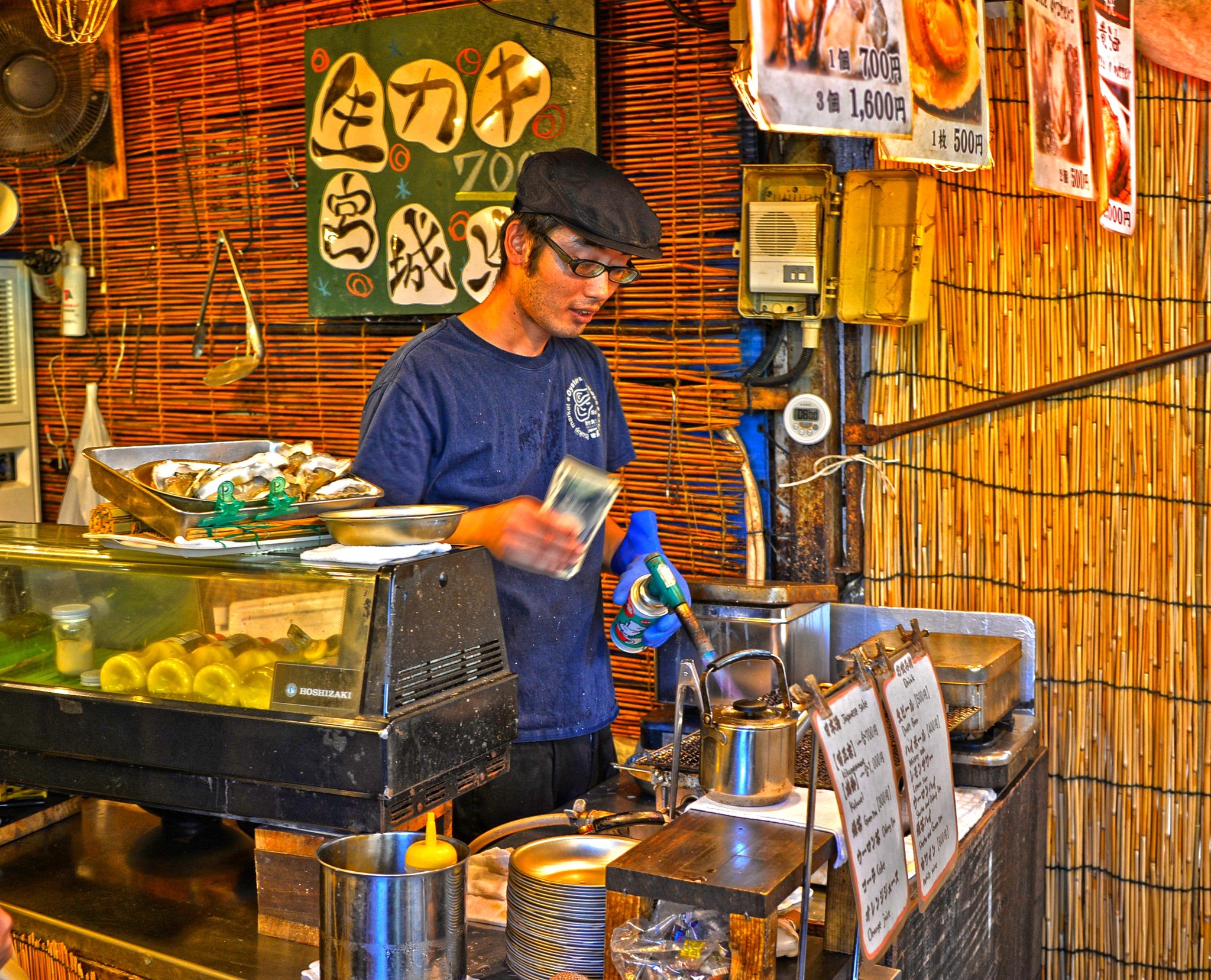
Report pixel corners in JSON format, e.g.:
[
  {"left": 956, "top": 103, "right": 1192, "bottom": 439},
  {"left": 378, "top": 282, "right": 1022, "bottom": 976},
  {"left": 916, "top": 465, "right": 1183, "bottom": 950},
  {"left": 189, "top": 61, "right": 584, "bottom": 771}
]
[{"left": 349, "top": 148, "right": 690, "bottom": 855}]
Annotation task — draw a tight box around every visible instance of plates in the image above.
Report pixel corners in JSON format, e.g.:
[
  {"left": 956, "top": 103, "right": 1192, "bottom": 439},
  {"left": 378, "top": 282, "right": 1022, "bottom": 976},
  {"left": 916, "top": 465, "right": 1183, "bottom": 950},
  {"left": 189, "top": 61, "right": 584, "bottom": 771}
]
[
  {"left": 133, "top": 459, "right": 274, "bottom": 510},
  {"left": 318, "top": 505, "right": 471, "bottom": 547},
  {"left": 503, "top": 835, "right": 642, "bottom": 979},
  {"left": 911, "top": 30, "right": 982, "bottom": 110}
]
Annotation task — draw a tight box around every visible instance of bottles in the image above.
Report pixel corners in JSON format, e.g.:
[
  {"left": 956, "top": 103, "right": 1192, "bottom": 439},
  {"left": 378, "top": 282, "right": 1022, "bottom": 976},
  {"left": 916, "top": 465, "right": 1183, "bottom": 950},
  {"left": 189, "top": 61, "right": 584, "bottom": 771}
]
[
  {"left": 194, "top": 638, "right": 298, "bottom": 706},
  {"left": 404, "top": 811, "right": 456, "bottom": 877},
  {"left": 237, "top": 651, "right": 308, "bottom": 707},
  {"left": 100, "top": 629, "right": 225, "bottom": 693},
  {"left": 61, "top": 251, "right": 88, "bottom": 336},
  {"left": 148, "top": 633, "right": 271, "bottom": 691}
]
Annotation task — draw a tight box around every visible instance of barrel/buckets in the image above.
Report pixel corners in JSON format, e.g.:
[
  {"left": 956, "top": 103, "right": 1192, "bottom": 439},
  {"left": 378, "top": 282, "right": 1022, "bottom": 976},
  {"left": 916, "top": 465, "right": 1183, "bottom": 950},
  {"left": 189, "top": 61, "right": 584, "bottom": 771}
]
[{"left": 314, "top": 831, "right": 473, "bottom": 980}]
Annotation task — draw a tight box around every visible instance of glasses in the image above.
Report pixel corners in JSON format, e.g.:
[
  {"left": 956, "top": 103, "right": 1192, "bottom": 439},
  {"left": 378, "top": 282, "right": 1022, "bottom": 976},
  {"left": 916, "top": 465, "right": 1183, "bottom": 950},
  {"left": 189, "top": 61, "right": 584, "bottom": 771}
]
[{"left": 528, "top": 223, "right": 642, "bottom": 284}]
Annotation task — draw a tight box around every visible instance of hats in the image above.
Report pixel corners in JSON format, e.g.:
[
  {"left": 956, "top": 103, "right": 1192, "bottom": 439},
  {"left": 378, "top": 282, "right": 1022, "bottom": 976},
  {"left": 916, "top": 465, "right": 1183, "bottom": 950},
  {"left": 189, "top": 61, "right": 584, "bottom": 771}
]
[{"left": 520, "top": 146, "right": 664, "bottom": 262}]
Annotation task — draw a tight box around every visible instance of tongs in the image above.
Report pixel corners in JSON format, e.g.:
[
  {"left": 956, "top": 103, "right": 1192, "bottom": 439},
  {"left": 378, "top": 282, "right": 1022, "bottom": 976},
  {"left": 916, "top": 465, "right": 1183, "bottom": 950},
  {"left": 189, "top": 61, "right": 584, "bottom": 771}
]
[{"left": 191, "top": 232, "right": 265, "bottom": 359}]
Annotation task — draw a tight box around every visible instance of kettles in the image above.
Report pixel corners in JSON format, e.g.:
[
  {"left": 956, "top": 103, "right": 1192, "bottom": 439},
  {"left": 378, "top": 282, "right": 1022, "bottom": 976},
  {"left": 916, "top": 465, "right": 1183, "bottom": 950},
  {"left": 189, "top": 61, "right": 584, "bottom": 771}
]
[{"left": 702, "top": 648, "right": 810, "bottom": 807}]
